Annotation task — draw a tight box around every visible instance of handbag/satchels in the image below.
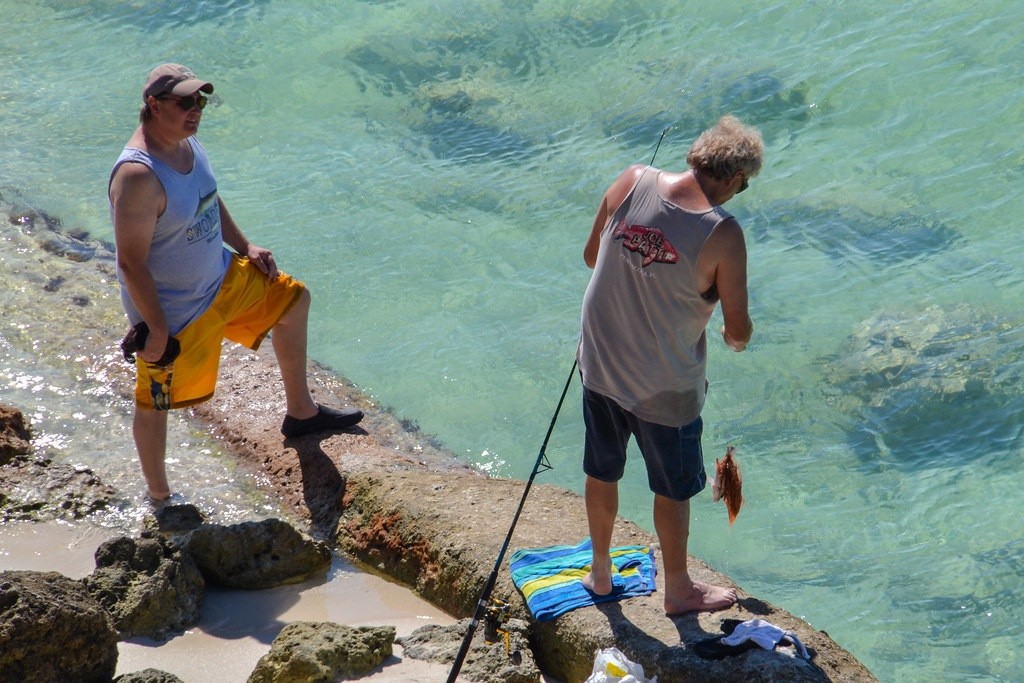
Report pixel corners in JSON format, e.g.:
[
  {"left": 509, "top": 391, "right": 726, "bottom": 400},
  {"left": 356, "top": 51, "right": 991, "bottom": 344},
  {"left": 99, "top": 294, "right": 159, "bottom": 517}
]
[{"left": 119, "top": 322, "right": 180, "bottom": 369}]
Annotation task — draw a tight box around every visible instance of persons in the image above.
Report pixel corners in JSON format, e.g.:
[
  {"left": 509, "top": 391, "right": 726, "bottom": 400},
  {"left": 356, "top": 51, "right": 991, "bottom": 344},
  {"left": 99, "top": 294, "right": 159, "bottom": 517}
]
[
  {"left": 577, "top": 115, "right": 765, "bottom": 615},
  {"left": 108, "top": 64, "right": 363, "bottom": 506}
]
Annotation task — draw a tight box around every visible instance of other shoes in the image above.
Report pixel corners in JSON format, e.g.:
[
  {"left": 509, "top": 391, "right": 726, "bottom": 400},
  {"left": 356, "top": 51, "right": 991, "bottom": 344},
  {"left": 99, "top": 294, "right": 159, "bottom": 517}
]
[
  {"left": 144, "top": 490, "right": 187, "bottom": 502},
  {"left": 281, "top": 403, "right": 363, "bottom": 436}
]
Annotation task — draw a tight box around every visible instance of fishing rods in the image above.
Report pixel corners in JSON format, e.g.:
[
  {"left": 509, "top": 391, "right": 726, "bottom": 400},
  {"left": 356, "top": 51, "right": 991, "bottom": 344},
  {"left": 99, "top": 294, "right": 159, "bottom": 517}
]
[{"left": 447, "top": 126, "right": 670, "bottom": 683}]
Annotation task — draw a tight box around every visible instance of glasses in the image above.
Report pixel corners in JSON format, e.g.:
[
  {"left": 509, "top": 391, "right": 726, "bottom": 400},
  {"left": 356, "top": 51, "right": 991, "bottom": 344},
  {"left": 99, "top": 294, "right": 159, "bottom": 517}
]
[
  {"left": 736, "top": 172, "right": 749, "bottom": 194},
  {"left": 162, "top": 96, "right": 208, "bottom": 110}
]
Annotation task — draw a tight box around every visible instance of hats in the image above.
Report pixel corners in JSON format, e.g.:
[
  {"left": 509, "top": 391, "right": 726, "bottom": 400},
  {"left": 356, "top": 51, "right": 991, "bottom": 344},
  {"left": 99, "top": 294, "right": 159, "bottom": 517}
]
[{"left": 143, "top": 63, "right": 214, "bottom": 97}]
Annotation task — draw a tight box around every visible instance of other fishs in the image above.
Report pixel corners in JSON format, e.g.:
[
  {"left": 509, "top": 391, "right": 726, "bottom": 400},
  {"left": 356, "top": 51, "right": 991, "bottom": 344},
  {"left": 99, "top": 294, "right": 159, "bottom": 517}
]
[{"left": 714, "top": 443, "right": 743, "bottom": 524}]
